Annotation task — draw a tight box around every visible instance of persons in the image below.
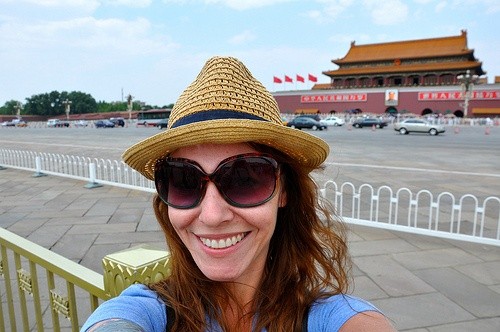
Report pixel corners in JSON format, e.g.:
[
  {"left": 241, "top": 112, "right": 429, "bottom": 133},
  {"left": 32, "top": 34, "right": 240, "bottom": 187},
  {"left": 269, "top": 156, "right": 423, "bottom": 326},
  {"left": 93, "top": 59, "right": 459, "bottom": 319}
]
[
  {"left": 485, "top": 116, "right": 493, "bottom": 135},
  {"left": 79, "top": 56, "right": 395, "bottom": 332}
]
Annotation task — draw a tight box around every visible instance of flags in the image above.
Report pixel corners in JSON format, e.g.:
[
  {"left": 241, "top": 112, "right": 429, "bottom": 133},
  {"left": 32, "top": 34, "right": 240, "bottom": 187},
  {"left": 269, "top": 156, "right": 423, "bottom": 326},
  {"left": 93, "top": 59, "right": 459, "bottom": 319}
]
[
  {"left": 297, "top": 76, "right": 304, "bottom": 82},
  {"left": 274, "top": 77, "right": 282, "bottom": 83},
  {"left": 309, "top": 74, "right": 317, "bottom": 82},
  {"left": 285, "top": 75, "right": 292, "bottom": 82}
]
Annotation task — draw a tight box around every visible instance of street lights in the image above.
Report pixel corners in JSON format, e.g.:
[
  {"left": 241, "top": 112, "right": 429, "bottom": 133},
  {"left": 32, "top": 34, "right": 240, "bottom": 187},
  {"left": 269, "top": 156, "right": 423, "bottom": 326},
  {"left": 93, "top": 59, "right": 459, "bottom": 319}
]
[
  {"left": 63, "top": 98, "right": 72, "bottom": 119},
  {"left": 456, "top": 70, "right": 486, "bottom": 119}
]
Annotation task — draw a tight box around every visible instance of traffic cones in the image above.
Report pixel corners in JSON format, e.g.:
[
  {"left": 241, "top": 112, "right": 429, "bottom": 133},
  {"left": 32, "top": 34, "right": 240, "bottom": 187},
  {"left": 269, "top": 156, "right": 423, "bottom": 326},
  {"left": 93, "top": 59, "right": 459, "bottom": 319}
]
[
  {"left": 486, "top": 127, "right": 491, "bottom": 135},
  {"left": 454, "top": 125, "right": 460, "bottom": 134},
  {"left": 372, "top": 124, "right": 377, "bottom": 132}
]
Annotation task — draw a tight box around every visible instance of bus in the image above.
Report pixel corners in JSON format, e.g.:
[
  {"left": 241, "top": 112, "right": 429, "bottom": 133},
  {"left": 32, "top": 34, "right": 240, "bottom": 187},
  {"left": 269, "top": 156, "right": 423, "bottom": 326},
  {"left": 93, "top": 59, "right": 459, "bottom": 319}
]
[{"left": 137, "top": 110, "right": 174, "bottom": 127}]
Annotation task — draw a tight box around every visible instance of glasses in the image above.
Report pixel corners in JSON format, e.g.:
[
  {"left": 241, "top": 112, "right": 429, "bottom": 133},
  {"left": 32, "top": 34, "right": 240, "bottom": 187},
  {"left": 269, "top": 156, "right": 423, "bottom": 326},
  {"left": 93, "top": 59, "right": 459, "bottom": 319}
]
[{"left": 154, "top": 153, "right": 283, "bottom": 210}]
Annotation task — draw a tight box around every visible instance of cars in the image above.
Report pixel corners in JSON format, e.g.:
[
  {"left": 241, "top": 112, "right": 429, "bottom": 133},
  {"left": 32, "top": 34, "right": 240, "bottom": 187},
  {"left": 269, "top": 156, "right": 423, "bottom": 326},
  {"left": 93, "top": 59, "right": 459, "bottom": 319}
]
[
  {"left": 353, "top": 118, "right": 388, "bottom": 129},
  {"left": 1, "top": 119, "right": 28, "bottom": 127},
  {"left": 46, "top": 119, "right": 69, "bottom": 128},
  {"left": 74, "top": 121, "right": 89, "bottom": 127},
  {"left": 95, "top": 117, "right": 126, "bottom": 129},
  {"left": 154, "top": 120, "right": 168, "bottom": 129},
  {"left": 286, "top": 115, "right": 345, "bottom": 131},
  {"left": 393, "top": 118, "right": 444, "bottom": 136}
]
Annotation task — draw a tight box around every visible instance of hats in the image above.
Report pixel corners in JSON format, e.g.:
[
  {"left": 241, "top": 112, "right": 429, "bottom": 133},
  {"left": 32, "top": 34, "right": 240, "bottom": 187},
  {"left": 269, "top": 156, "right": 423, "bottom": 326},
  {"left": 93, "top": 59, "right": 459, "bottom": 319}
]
[{"left": 122, "top": 57, "right": 329, "bottom": 181}]
